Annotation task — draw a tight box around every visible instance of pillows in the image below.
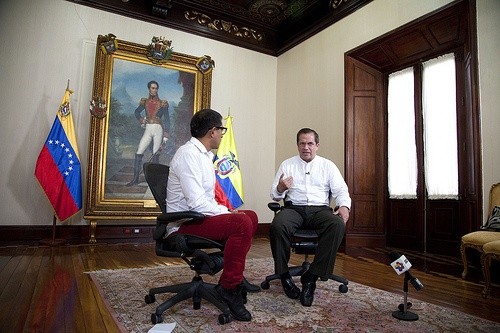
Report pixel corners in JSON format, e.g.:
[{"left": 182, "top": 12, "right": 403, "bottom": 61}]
[{"left": 480, "top": 206, "right": 500, "bottom": 232}]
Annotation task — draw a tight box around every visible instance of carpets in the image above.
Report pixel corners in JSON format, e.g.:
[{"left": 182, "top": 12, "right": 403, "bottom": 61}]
[{"left": 84, "top": 256, "right": 500, "bottom": 333}]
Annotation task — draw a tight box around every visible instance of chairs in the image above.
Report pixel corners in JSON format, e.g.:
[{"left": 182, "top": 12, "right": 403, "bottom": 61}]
[
  {"left": 480, "top": 240, "right": 500, "bottom": 300},
  {"left": 142, "top": 162, "right": 248, "bottom": 325},
  {"left": 261, "top": 190, "right": 349, "bottom": 293},
  {"left": 459, "top": 183, "right": 500, "bottom": 280}
]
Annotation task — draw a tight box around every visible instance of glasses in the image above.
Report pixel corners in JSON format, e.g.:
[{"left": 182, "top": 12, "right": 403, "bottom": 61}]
[{"left": 211, "top": 126, "right": 227, "bottom": 135}]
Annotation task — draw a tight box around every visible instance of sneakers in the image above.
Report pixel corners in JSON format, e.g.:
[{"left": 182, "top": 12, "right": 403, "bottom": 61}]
[
  {"left": 280, "top": 274, "right": 300, "bottom": 299},
  {"left": 301, "top": 272, "right": 316, "bottom": 306}
]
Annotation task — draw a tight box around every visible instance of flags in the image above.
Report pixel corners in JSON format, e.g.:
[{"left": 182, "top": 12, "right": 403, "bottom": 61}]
[
  {"left": 213, "top": 115, "right": 245, "bottom": 212},
  {"left": 35, "top": 89, "right": 83, "bottom": 223}
]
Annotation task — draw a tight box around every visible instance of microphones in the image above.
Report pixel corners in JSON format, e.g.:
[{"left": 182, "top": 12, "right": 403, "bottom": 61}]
[{"left": 389, "top": 252, "right": 424, "bottom": 291}]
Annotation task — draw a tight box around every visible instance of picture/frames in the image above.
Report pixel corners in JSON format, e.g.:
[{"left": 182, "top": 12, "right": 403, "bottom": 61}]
[{"left": 82, "top": 35, "right": 215, "bottom": 221}]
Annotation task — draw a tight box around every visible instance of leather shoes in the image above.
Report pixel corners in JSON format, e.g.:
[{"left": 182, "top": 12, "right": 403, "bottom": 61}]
[
  {"left": 241, "top": 276, "right": 262, "bottom": 294},
  {"left": 214, "top": 282, "right": 252, "bottom": 321}
]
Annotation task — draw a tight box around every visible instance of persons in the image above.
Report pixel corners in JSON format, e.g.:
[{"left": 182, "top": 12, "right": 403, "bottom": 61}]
[
  {"left": 269, "top": 128, "right": 352, "bottom": 308},
  {"left": 165, "top": 109, "right": 261, "bottom": 322}
]
